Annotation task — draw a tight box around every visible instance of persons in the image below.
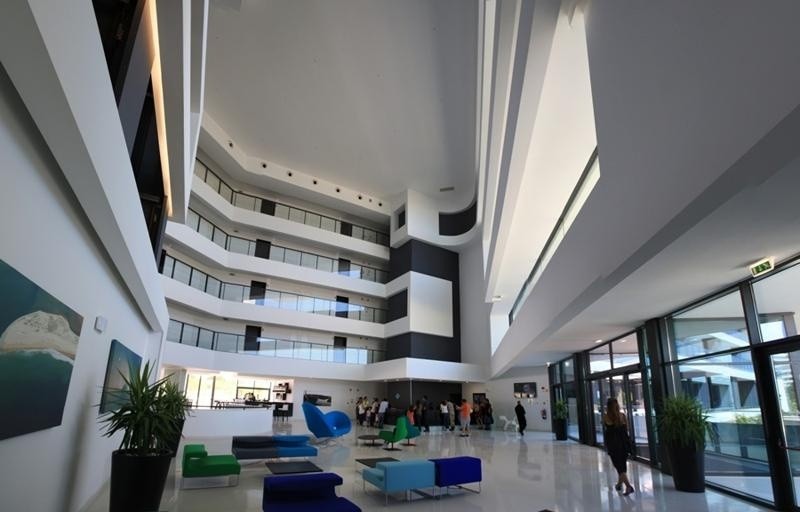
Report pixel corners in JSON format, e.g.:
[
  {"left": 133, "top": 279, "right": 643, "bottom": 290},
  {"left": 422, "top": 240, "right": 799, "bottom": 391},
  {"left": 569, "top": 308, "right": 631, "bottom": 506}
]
[
  {"left": 601, "top": 397, "right": 634, "bottom": 495},
  {"left": 514, "top": 400, "right": 527, "bottom": 435},
  {"left": 355, "top": 395, "right": 389, "bottom": 429},
  {"left": 407, "top": 395, "right": 494, "bottom": 436}
]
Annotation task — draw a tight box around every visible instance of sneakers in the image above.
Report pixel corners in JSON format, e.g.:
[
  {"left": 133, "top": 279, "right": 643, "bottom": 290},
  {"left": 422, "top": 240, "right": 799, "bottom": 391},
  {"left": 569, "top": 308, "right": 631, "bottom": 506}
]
[
  {"left": 615, "top": 484, "right": 621, "bottom": 491},
  {"left": 623, "top": 486, "right": 634, "bottom": 497}
]
